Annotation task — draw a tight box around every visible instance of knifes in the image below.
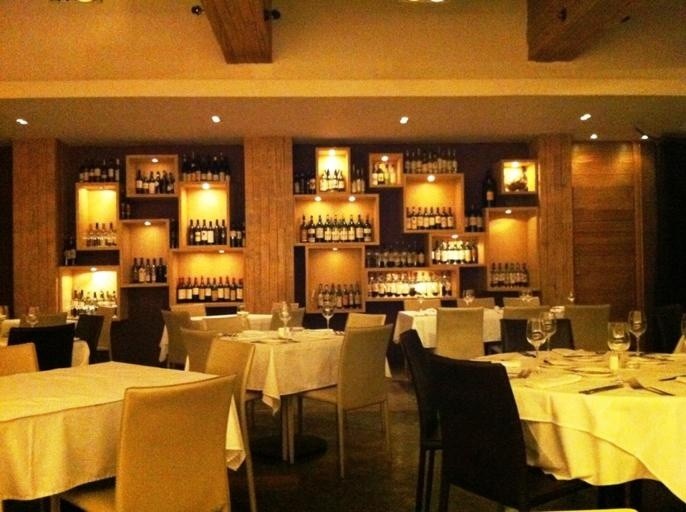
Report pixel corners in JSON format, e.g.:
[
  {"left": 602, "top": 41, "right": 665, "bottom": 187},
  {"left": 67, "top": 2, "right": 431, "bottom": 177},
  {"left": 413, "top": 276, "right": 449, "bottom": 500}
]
[{"left": 658, "top": 375, "right": 685, "bottom": 382}]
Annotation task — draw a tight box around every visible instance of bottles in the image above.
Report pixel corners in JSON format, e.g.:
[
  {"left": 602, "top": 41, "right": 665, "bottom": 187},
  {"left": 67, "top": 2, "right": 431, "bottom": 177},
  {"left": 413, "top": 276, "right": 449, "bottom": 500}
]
[
  {"left": 370, "top": 270, "right": 453, "bottom": 297},
  {"left": 429, "top": 240, "right": 477, "bottom": 266},
  {"left": 422, "top": 203, "right": 454, "bottom": 230},
  {"left": 65, "top": 150, "right": 245, "bottom": 315},
  {"left": 290, "top": 155, "right": 373, "bottom": 310},
  {"left": 369, "top": 160, "right": 428, "bottom": 267},
  {"left": 403, "top": 146, "right": 458, "bottom": 172},
  {"left": 488, "top": 262, "right": 529, "bottom": 287},
  {"left": 463, "top": 203, "right": 485, "bottom": 233},
  {"left": 480, "top": 166, "right": 497, "bottom": 208}
]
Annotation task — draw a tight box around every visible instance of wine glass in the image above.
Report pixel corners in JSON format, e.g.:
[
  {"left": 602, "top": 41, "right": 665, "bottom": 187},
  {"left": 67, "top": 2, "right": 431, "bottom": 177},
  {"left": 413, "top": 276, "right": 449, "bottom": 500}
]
[
  {"left": 413, "top": 291, "right": 428, "bottom": 316},
  {"left": 518, "top": 289, "right": 576, "bottom": 309},
  {"left": 319, "top": 299, "right": 335, "bottom": 337},
  {"left": 523, "top": 312, "right": 646, "bottom": 381},
  {"left": 275, "top": 301, "right": 294, "bottom": 337},
  {"left": 462, "top": 288, "right": 474, "bottom": 308}
]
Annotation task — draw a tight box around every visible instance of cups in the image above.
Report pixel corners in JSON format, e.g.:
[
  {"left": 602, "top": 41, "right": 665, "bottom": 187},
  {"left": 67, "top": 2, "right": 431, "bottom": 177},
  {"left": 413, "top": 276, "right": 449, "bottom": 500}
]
[
  {"left": 24, "top": 305, "right": 41, "bottom": 327},
  {"left": 680, "top": 313, "right": 686, "bottom": 346},
  {"left": 0, "top": 305, "right": 9, "bottom": 323}
]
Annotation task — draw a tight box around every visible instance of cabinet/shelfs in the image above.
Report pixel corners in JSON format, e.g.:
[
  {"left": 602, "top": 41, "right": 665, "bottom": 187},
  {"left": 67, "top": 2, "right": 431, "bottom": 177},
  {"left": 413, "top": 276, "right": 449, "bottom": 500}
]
[
  {"left": 290, "top": 143, "right": 540, "bottom": 311},
  {"left": 60, "top": 145, "right": 244, "bottom": 317}
]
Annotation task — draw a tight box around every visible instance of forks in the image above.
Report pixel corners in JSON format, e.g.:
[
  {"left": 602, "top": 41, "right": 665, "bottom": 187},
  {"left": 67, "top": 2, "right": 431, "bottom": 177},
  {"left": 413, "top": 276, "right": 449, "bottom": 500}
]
[{"left": 624, "top": 375, "right": 666, "bottom": 396}]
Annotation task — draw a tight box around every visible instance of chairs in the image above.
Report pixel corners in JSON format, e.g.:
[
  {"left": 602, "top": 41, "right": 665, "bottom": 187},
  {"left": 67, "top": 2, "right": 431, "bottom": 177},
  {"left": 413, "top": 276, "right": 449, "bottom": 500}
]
[{"left": 0, "top": 286, "right": 686, "bottom": 512}]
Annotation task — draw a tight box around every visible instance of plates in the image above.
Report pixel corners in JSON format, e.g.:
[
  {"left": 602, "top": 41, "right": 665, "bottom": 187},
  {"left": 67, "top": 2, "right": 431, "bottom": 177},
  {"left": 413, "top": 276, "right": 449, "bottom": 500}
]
[{"left": 525, "top": 374, "right": 580, "bottom": 389}]
